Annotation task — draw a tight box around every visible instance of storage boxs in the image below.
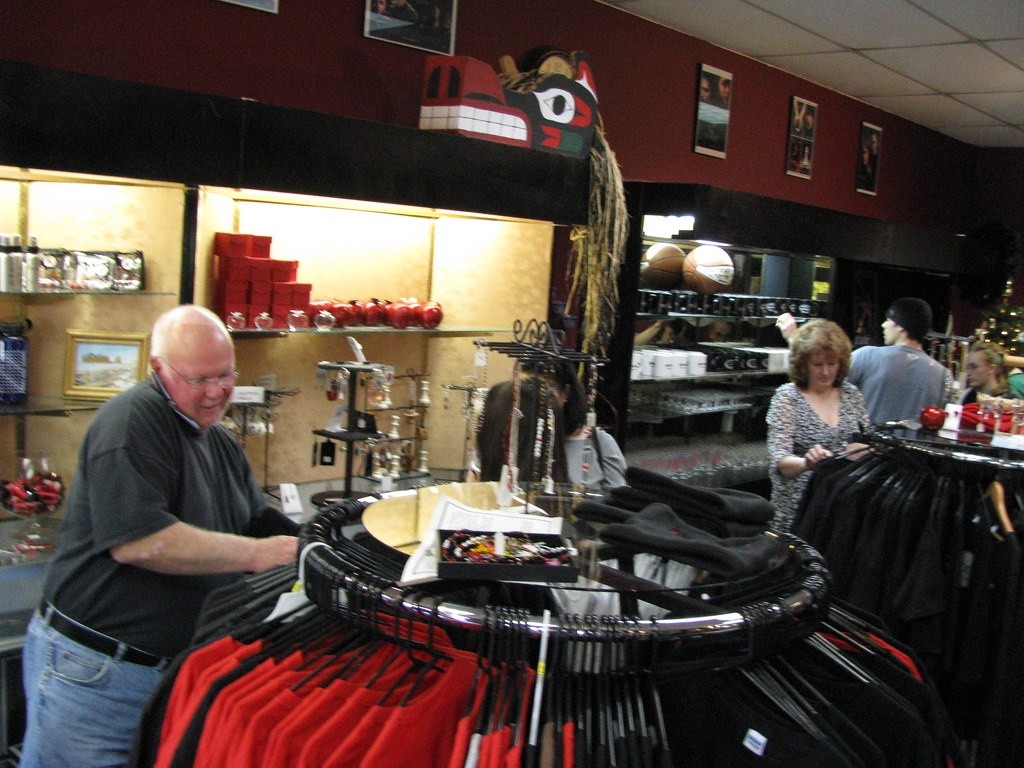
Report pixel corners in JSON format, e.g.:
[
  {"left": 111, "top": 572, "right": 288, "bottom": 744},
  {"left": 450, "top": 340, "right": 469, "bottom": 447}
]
[
  {"left": 216, "top": 234, "right": 312, "bottom": 329},
  {"left": 437, "top": 530, "right": 580, "bottom": 584}
]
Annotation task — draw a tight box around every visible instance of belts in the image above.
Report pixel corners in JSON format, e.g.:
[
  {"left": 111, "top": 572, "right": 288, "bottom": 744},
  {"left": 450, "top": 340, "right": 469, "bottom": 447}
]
[{"left": 40, "top": 598, "right": 171, "bottom": 671}]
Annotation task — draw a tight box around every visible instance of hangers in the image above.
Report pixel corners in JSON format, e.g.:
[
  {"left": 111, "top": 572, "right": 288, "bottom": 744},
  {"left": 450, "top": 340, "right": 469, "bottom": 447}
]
[{"left": 230, "top": 429, "right": 1024, "bottom": 768}]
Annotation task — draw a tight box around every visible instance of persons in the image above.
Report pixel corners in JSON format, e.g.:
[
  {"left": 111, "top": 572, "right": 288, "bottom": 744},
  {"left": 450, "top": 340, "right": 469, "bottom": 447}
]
[
  {"left": 371, "top": 0, "right": 420, "bottom": 22},
  {"left": 955, "top": 339, "right": 1024, "bottom": 405},
  {"left": 791, "top": 102, "right": 814, "bottom": 173},
  {"left": 765, "top": 317, "right": 872, "bottom": 533},
  {"left": 699, "top": 72, "right": 731, "bottom": 110},
  {"left": 634, "top": 317, "right": 786, "bottom": 348},
  {"left": 859, "top": 134, "right": 878, "bottom": 187},
  {"left": 17, "top": 303, "right": 299, "bottom": 768},
  {"left": 464, "top": 362, "right": 630, "bottom": 496},
  {"left": 775, "top": 296, "right": 953, "bottom": 439}
]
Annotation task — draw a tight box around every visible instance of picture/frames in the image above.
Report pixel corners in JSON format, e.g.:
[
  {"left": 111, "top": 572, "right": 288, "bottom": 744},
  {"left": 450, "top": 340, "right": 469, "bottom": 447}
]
[{"left": 59, "top": 329, "right": 150, "bottom": 404}]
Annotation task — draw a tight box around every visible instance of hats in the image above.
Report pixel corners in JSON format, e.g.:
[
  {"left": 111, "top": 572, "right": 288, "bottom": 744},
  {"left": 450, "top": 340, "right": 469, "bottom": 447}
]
[{"left": 888, "top": 297, "right": 931, "bottom": 335}]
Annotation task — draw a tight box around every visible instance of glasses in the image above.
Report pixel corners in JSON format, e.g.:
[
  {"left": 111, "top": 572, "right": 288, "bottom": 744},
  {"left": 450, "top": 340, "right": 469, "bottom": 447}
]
[{"left": 157, "top": 355, "right": 240, "bottom": 389}]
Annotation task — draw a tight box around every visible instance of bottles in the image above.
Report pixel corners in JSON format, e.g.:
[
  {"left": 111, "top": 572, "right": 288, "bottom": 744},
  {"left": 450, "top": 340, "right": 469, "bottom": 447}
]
[
  {"left": 0, "top": 233, "right": 39, "bottom": 293},
  {"left": 0, "top": 321, "right": 29, "bottom": 405}
]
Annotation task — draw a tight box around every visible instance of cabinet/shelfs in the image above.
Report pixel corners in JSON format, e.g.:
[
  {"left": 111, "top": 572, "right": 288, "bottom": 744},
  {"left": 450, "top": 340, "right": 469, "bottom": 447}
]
[
  {"left": 637, "top": 236, "right": 835, "bottom": 442},
  {"left": 229, "top": 325, "right": 531, "bottom": 527},
  {"left": 0, "top": 288, "right": 176, "bottom": 648}
]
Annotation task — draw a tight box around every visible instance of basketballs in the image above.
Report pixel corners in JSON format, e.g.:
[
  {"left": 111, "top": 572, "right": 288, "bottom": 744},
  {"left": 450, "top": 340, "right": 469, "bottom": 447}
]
[
  {"left": 641, "top": 243, "right": 686, "bottom": 290},
  {"left": 684, "top": 245, "right": 735, "bottom": 294}
]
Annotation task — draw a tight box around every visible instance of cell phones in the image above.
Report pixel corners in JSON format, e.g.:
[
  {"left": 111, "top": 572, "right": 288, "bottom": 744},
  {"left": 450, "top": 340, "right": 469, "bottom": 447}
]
[{"left": 149, "top": 369, "right": 202, "bottom": 436}]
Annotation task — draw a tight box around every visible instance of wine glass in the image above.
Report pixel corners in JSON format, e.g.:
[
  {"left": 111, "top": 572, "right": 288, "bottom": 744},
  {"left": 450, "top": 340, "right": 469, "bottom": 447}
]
[{"left": 0, "top": 449, "right": 66, "bottom": 553}]
